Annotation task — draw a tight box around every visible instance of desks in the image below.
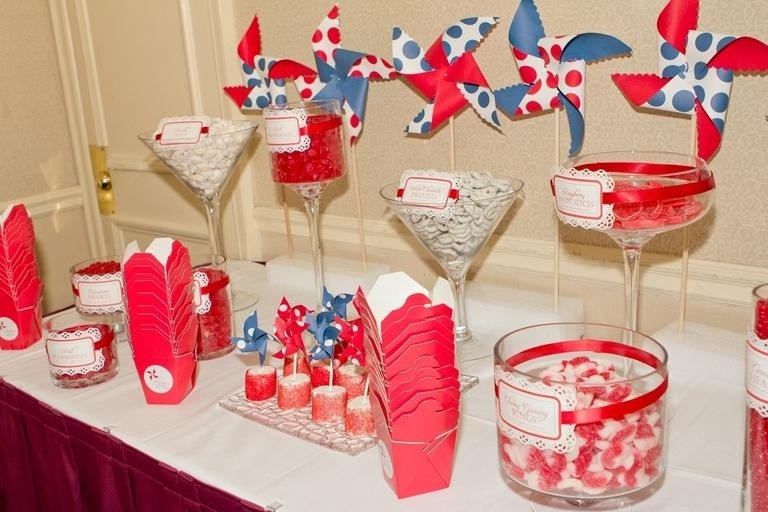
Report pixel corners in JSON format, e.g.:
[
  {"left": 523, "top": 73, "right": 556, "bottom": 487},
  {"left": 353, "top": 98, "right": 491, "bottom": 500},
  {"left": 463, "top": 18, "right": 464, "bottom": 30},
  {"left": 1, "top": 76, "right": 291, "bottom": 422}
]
[{"left": 1, "top": 253, "right": 754, "bottom": 510}]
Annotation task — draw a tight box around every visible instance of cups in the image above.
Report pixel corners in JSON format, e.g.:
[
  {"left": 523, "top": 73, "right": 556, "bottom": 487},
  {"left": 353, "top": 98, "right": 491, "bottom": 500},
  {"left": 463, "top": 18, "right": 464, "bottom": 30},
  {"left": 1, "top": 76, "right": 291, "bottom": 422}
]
[
  {"left": 186, "top": 254, "right": 237, "bottom": 362},
  {"left": 493, "top": 323, "right": 668, "bottom": 511},
  {"left": 45, "top": 256, "right": 127, "bottom": 389}
]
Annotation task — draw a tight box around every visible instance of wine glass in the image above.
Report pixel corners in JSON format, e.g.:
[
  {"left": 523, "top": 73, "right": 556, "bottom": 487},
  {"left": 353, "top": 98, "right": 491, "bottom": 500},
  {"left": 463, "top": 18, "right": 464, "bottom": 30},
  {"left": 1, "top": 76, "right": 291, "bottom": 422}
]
[
  {"left": 554, "top": 150, "right": 716, "bottom": 366},
  {"left": 136, "top": 119, "right": 260, "bottom": 312},
  {"left": 380, "top": 177, "right": 524, "bottom": 364},
  {"left": 262, "top": 100, "right": 351, "bottom": 310}
]
